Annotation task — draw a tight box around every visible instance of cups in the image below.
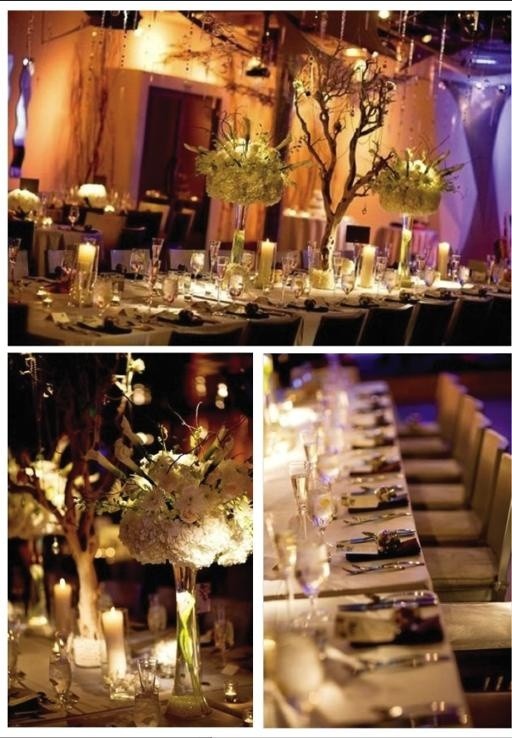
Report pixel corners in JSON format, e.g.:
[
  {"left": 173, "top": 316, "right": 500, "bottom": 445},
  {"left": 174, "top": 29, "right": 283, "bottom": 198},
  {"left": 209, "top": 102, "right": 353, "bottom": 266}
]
[{"left": 375, "top": 256, "right": 387, "bottom": 282}]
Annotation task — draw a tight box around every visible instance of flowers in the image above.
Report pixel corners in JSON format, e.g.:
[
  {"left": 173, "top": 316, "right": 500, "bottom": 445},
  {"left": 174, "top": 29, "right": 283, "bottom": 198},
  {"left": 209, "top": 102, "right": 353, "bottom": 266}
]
[
  {"left": 8, "top": 452, "right": 83, "bottom": 539},
  {"left": 187, "top": 105, "right": 312, "bottom": 207},
  {"left": 367, "top": 138, "right": 470, "bottom": 216},
  {"left": 87, "top": 410, "right": 253, "bottom": 567}
]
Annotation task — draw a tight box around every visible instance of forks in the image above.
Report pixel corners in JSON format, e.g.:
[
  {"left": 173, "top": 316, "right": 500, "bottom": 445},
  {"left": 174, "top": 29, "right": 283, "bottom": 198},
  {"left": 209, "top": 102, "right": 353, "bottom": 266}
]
[{"left": 342, "top": 560, "right": 414, "bottom": 574}]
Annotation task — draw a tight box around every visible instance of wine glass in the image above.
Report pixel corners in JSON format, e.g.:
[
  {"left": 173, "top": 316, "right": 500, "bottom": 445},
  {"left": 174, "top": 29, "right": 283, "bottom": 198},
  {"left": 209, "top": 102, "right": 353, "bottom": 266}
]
[
  {"left": 55, "top": 632, "right": 78, "bottom": 700},
  {"left": 281, "top": 252, "right": 295, "bottom": 301},
  {"left": 210, "top": 239, "right": 220, "bottom": 280},
  {"left": 138, "top": 657, "right": 157, "bottom": 692},
  {"left": 160, "top": 271, "right": 178, "bottom": 311},
  {"left": 130, "top": 246, "right": 149, "bottom": 280},
  {"left": 341, "top": 268, "right": 355, "bottom": 300},
  {"left": 332, "top": 254, "right": 344, "bottom": 294},
  {"left": 217, "top": 606, "right": 229, "bottom": 661},
  {"left": 487, "top": 255, "right": 495, "bottom": 288},
  {"left": 190, "top": 253, "right": 206, "bottom": 286},
  {"left": 149, "top": 260, "right": 160, "bottom": 297},
  {"left": 48, "top": 653, "right": 72, "bottom": 718},
  {"left": 351, "top": 240, "right": 361, "bottom": 265},
  {"left": 151, "top": 237, "right": 165, "bottom": 278},
  {"left": 131, "top": 687, "right": 160, "bottom": 726},
  {"left": 230, "top": 275, "right": 244, "bottom": 307},
  {"left": 216, "top": 255, "right": 228, "bottom": 285},
  {"left": 264, "top": 382, "right": 348, "bottom": 729},
  {"left": 452, "top": 255, "right": 463, "bottom": 284}
]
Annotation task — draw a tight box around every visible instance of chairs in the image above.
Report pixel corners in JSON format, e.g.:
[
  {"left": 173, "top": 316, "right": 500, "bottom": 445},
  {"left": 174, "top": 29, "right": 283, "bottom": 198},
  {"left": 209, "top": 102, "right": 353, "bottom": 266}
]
[
  {"left": 7, "top": 599, "right": 253, "bottom": 727},
  {"left": 7, "top": 567, "right": 253, "bottom": 660}
]
